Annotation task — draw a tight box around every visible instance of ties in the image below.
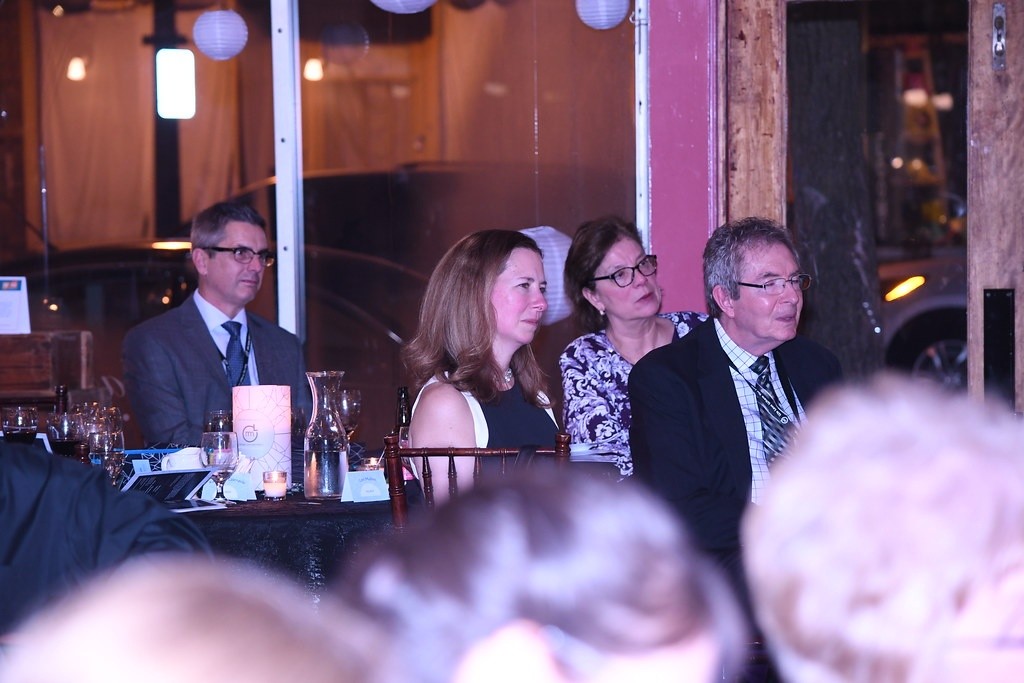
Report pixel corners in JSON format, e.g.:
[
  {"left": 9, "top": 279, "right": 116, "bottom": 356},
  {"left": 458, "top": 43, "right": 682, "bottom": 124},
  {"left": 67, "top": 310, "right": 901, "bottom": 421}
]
[
  {"left": 749, "top": 356, "right": 795, "bottom": 473},
  {"left": 221, "top": 321, "right": 251, "bottom": 386}
]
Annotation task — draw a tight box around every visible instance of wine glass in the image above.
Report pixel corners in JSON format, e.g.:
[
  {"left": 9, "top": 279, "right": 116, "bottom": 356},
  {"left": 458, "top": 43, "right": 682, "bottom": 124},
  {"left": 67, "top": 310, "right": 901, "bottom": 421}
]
[{"left": 200, "top": 431, "right": 239, "bottom": 506}]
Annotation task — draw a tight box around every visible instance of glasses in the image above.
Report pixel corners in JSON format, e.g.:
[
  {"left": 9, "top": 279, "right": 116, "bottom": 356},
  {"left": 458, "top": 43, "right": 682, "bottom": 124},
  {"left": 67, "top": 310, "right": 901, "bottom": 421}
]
[
  {"left": 591, "top": 255, "right": 659, "bottom": 287},
  {"left": 730, "top": 274, "right": 812, "bottom": 295},
  {"left": 202, "top": 247, "right": 274, "bottom": 266}
]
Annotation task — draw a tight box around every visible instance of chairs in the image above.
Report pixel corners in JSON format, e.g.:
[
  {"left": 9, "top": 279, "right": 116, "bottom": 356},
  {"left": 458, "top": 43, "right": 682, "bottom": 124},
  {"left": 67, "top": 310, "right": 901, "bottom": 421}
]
[{"left": 383, "top": 384, "right": 571, "bottom": 534}]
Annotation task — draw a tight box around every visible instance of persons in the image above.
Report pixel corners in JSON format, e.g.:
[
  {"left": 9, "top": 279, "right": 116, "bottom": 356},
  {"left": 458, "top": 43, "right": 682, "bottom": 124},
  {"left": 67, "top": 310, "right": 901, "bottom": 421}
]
[
  {"left": 0, "top": 440, "right": 213, "bottom": 633},
  {"left": 627, "top": 216, "right": 842, "bottom": 683},
  {"left": 559, "top": 217, "right": 708, "bottom": 478},
  {"left": 123, "top": 197, "right": 311, "bottom": 449},
  {"left": 400, "top": 229, "right": 561, "bottom": 504},
  {"left": 0, "top": 550, "right": 411, "bottom": 682},
  {"left": 351, "top": 459, "right": 752, "bottom": 683},
  {"left": 745, "top": 366, "right": 1023, "bottom": 683}
]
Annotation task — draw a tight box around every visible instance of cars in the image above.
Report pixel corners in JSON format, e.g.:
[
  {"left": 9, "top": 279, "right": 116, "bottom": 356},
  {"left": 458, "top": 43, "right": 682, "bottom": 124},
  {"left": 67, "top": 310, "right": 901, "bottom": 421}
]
[
  {"left": 0, "top": 231, "right": 429, "bottom": 455},
  {"left": 876, "top": 252, "right": 1015, "bottom": 408}
]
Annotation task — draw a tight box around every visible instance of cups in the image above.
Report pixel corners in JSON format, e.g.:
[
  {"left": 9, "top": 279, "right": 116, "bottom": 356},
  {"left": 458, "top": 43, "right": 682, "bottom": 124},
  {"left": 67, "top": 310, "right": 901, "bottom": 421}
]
[
  {"left": 208, "top": 410, "right": 233, "bottom": 433},
  {"left": 2, "top": 402, "right": 125, "bottom": 485},
  {"left": 291, "top": 407, "right": 308, "bottom": 449},
  {"left": 263, "top": 470, "right": 287, "bottom": 502}
]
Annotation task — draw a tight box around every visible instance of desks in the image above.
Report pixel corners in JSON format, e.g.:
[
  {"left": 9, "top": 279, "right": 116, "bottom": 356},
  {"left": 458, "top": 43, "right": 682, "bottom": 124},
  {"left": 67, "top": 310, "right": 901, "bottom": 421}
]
[{"left": 183, "top": 496, "right": 393, "bottom": 592}]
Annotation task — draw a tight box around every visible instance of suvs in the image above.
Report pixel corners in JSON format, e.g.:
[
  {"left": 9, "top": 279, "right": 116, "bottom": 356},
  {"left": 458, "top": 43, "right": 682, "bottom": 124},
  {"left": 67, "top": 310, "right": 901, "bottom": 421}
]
[{"left": 182, "top": 159, "right": 619, "bottom": 287}]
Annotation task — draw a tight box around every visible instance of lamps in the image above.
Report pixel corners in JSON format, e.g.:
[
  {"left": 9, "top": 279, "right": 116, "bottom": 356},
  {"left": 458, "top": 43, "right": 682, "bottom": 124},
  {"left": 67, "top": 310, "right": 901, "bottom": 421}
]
[{"left": 232, "top": 385, "right": 291, "bottom": 491}]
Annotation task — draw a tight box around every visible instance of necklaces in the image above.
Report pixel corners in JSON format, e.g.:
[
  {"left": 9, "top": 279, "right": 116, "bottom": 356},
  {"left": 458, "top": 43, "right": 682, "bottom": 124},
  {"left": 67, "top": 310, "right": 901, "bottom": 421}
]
[{"left": 504, "top": 368, "right": 512, "bottom": 383}]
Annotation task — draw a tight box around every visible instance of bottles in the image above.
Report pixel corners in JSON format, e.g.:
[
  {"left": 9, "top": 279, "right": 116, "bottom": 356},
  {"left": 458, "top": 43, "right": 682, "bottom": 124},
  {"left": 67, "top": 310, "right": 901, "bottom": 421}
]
[
  {"left": 53, "top": 383, "right": 69, "bottom": 414},
  {"left": 304, "top": 370, "right": 349, "bottom": 501},
  {"left": 336, "top": 389, "right": 362, "bottom": 438},
  {"left": 391, "top": 386, "right": 421, "bottom": 502}
]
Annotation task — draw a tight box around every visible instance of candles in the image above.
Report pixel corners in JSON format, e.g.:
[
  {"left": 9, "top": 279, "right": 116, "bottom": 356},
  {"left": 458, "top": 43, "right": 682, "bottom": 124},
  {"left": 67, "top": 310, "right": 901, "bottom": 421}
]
[
  {"left": 263, "top": 470, "right": 289, "bottom": 497},
  {"left": 309, "top": 452, "right": 317, "bottom": 496}
]
